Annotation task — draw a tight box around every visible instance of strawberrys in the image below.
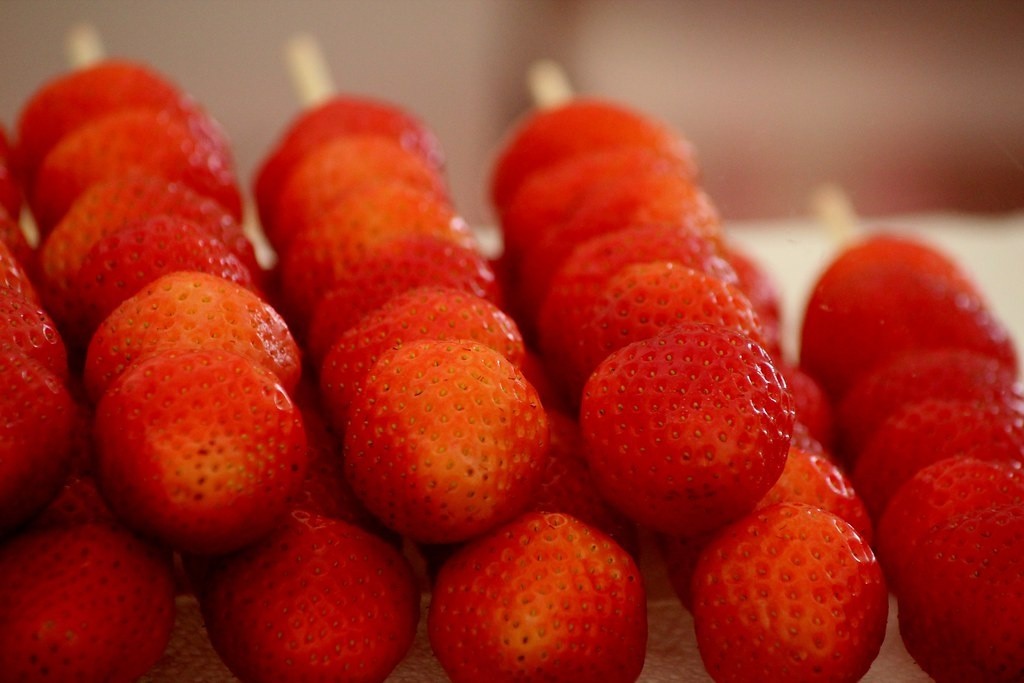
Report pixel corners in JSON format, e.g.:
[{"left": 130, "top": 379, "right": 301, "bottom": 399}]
[{"left": 1, "top": 61, "right": 1024, "bottom": 680}]
